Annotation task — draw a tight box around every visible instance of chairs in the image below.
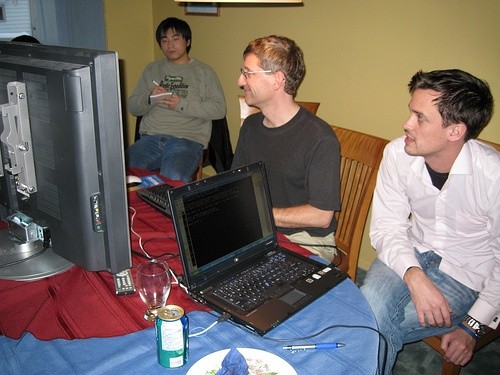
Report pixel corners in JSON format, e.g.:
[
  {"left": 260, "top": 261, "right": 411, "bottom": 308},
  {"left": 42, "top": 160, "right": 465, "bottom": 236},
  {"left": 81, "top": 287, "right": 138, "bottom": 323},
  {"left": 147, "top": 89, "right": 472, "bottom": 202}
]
[
  {"left": 237, "top": 94, "right": 321, "bottom": 130},
  {"left": 192, "top": 146, "right": 209, "bottom": 182},
  {"left": 331, "top": 125, "right": 391, "bottom": 284},
  {"left": 420, "top": 138, "right": 500, "bottom": 375}
]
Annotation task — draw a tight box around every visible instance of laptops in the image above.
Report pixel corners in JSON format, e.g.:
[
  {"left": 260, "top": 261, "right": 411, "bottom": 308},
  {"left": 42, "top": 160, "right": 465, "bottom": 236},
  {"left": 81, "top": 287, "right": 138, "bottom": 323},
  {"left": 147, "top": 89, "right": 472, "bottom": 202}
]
[{"left": 167, "top": 160, "right": 349, "bottom": 337}]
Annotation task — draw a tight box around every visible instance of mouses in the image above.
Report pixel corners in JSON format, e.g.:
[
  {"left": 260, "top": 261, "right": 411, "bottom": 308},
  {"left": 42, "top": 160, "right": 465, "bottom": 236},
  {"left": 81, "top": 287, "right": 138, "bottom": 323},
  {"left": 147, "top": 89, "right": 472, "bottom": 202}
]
[{"left": 126, "top": 176, "right": 142, "bottom": 187}]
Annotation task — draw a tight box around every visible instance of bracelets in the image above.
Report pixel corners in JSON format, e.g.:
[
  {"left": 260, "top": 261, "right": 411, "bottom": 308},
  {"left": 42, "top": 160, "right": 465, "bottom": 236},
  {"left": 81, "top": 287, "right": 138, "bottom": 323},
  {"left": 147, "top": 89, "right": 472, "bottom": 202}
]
[{"left": 458, "top": 323, "right": 480, "bottom": 341}]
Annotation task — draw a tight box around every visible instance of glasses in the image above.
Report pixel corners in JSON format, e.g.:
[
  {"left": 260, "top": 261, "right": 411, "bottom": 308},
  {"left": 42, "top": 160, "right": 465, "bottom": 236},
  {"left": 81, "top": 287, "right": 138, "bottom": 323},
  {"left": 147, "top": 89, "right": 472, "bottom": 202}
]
[{"left": 241, "top": 68, "right": 272, "bottom": 77}]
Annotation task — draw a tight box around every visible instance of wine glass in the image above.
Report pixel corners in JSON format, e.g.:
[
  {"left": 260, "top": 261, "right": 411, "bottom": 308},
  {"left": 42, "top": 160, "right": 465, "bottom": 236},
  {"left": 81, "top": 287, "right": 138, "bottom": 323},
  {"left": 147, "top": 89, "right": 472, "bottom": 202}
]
[{"left": 136, "top": 260, "right": 171, "bottom": 321}]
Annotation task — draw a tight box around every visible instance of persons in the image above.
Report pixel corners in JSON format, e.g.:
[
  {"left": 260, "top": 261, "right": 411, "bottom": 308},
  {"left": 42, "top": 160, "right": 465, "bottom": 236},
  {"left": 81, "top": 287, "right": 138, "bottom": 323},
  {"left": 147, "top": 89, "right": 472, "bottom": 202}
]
[
  {"left": 231, "top": 35, "right": 342, "bottom": 265},
  {"left": 359, "top": 68, "right": 500, "bottom": 375},
  {"left": 125, "top": 17, "right": 227, "bottom": 184}
]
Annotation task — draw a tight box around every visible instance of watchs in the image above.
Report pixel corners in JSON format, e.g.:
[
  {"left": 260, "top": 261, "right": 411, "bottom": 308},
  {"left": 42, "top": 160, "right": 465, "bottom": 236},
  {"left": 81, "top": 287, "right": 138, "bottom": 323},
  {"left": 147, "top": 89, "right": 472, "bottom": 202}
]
[{"left": 462, "top": 314, "right": 487, "bottom": 337}]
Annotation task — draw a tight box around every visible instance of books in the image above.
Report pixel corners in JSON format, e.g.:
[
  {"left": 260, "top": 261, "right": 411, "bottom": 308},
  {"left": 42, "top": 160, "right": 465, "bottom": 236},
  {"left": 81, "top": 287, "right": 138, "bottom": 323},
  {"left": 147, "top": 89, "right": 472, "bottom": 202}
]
[{"left": 148, "top": 92, "right": 172, "bottom": 104}]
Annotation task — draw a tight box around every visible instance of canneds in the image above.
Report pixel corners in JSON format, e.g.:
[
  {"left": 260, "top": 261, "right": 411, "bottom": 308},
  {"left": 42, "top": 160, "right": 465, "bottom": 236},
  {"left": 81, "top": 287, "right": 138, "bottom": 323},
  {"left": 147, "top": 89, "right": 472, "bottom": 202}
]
[{"left": 154, "top": 304, "right": 189, "bottom": 369}]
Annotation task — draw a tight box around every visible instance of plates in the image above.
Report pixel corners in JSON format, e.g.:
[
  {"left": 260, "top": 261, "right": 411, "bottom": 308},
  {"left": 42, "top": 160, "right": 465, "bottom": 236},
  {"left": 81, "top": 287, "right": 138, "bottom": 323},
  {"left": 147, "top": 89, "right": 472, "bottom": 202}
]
[{"left": 186, "top": 347, "right": 298, "bottom": 375}]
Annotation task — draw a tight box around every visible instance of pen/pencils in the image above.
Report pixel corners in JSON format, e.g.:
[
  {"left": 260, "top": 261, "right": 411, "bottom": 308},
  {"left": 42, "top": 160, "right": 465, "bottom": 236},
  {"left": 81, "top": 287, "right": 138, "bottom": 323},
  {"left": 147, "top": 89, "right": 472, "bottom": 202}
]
[
  {"left": 282, "top": 342, "right": 347, "bottom": 350},
  {"left": 151, "top": 79, "right": 163, "bottom": 87}
]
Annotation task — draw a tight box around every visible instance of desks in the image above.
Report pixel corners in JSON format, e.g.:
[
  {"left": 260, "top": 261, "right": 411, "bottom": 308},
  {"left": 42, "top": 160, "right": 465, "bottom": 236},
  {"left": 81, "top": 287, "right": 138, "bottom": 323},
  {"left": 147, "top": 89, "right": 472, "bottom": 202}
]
[{"left": 1, "top": 165, "right": 380, "bottom": 374}]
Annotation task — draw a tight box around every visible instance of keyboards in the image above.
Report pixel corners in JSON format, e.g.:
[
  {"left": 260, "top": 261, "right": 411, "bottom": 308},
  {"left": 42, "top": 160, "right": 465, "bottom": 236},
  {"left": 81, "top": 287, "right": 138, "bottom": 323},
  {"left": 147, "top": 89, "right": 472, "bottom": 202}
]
[{"left": 136, "top": 183, "right": 178, "bottom": 219}]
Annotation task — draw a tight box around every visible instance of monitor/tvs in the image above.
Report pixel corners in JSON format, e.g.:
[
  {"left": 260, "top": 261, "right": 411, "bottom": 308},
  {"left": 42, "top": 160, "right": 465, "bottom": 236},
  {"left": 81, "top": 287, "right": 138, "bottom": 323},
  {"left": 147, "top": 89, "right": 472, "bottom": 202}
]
[{"left": 0, "top": 40, "right": 131, "bottom": 282}]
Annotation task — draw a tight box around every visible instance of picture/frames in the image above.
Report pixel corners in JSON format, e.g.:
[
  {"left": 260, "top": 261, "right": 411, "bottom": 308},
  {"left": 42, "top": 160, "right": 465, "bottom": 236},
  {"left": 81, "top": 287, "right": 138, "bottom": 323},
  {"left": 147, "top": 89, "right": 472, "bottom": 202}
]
[{"left": 183, "top": 2, "right": 221, "bottom": 17}]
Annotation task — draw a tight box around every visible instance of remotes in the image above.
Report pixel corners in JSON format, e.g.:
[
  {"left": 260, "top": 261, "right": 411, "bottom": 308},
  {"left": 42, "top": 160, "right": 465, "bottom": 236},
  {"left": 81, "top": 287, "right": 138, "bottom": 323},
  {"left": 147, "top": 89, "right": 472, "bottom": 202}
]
[{"left": 113, "top": 268, "right": 136, "bottom": 296}]
